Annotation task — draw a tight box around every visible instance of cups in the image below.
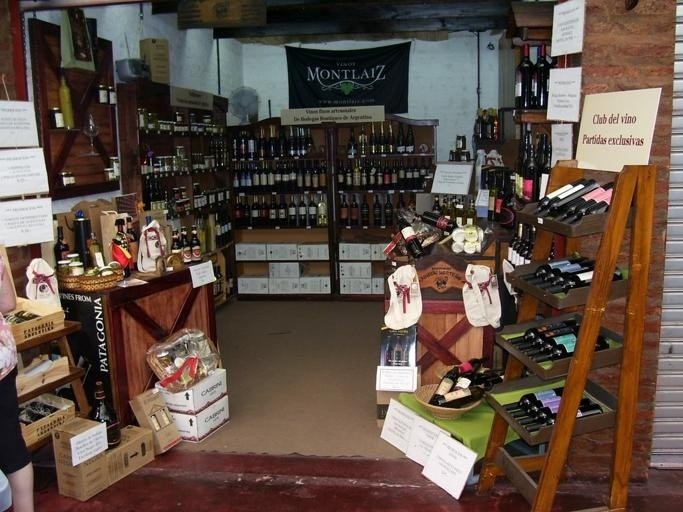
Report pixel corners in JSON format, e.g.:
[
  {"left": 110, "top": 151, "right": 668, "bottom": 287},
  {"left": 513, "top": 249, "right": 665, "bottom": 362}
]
[
  {"left": 57, "top": 260, "right": 71, "bottom": 271},
  {"left": 67, "top": 254, "right": 80, "bottom": 262},
  {"left": 69, "top": 263, "right": 84, "bottom": 277}
]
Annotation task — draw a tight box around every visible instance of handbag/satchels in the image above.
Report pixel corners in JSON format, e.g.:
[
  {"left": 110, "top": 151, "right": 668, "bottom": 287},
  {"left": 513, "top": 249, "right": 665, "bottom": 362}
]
[
  {"left": 100, "top": 210, "right": 129, "bottom": 269},
  {"left": 463, "top": 263, "right": 502, "bottom": 329},
  {"left": 72, "top": 200, "right": 113, "bottom": 246},
  {"left": 384, "top": 265, "right": 423, "bottom": 330},
  {"left": 25, "top": 258, "right": 60, "bottom": 307},
  {"left": 137, "top": 220, "right": 166, "bottom": 272}
]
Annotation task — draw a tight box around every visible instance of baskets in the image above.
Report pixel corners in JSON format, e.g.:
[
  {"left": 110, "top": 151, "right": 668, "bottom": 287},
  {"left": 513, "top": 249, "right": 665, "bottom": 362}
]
[
  {"left": 5, "top": 297, "right": 65, "bottom": 345},
  {"left": 19, "top": 393, "right": 76, "bottom": 447},
  {"left": 81, "top": 272, "right": 117, "bottom": 292},
  {"left": 114, "top": 266, "right": 124, "bottom": 282},
  {"left": 56, "top": 269, "right": 81, "bottom": 289}
]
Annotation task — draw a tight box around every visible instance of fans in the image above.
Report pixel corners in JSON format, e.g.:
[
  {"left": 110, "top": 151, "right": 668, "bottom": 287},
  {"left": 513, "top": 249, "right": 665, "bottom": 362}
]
[{"left": 230, "top": 86, "right": 259, "bottom": 125}]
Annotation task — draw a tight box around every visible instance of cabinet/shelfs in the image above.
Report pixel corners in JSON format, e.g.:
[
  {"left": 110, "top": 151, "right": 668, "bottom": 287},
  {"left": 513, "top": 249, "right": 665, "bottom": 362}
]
[
  {"left": 476, "top": 161, "right": 657, "bottom": 512},
  {"left": 332, "top": 114, "right": 438, "bottom": 301},
  {"left": 498, "top": 7, "right": 569, "bottom": 295},
  {"left": 4, "top": 306, "right": 93, "bottom": 470},
  {"left": 232, "top": 118, "right": 334, "bottom": 301},
  {"left": 119, "top": 81, "right": 232, "bottom": 314},
  {"left": 27, "top": 18, "right": 121, "bottom": 201}
]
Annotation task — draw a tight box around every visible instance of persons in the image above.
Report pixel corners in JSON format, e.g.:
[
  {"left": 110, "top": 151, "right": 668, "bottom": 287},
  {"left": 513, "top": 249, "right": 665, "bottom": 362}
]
[{"left": 0, "top": 249, "right": 35, "bottom": 512}]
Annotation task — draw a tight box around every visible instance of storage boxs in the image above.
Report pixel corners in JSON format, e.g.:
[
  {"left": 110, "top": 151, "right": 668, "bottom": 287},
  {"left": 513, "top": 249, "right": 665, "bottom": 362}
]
[
  {"left": 140, "top": 38, "right": 170, "bottom": 84},
  {"left": 51, "top": 417, "right": 155, "bottom": 503},
  {"left": 377, "top": 366, "right": 422, "bottom": 429},
  {"left": 163, "top": 394, "right": 230, "bottom": 443},
  {"left": 154, "top": 368, "right": 227, "bottom": 414}
]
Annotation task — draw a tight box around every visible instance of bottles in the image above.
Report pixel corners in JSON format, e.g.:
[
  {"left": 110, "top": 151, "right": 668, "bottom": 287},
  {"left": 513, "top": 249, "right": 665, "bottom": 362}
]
[
  {"left": 385, "top": 195, "right": 394, "bottom": 225},
  {"left": 29, "top": 402, "right": 58, "bottom": 415},
  {"left": 552, "top": 182, "right": 614, "bottom": 223},
  {"left": 18, "top": 407, "right": 43, "bottom": 422},
  {"left": 319, "top": 161, "right": 327, "bottom": 187},
  {"left": 288, "top": 194, "right": 296, "bottom": 226},
  {"left": 506, "top": 224, "right": 536, "bottom": 267},
  {"left": 507, "top": 320, "right": 577, "bottom": 345},
  {"left": 145, "top": 216, "right": 153, "bottom": 228},
  {"left": 420, "top": 157, "right": 428, "bottom": 189},
  {"left": 482, "top": 171, "right": 488, "bottom": 189},
  {"left": 232, "top": 130, "right": 239, "bottom": 162},
  {"left": 198, "top": 216, "right": 210, "bottom": 254},
  {"left": 448, "top": 355, "right": 490, "bottom": 375},
  {"left": 384, "top": 336, "right": 392, "bottom": 366},
  {"left": 17, "top": 418, "right": 30, "bottom": 427},
  {"left": 384, "top": 159, "right": 390, "bottom": 189},
  {"left": 509, "top": 395, "right": 563, "bottom": 418},
  {"left": 387, "top": 120, "right": 395, "bottom": 154},
  {"left": 87, "top": 232, "right": 105, "bottom": 268},
  {"left": 318, "top": 145, "right": 325, "bottom": 154},
  {"left": 142, "top": 108, "right": 148, "bottom": 133},
  {"left": 560, "top": 184, "right": 616, "bottom": 225},
  {"left": 354, "top": 159, "right": 361, "bottom": 189},
  {"left": 345, "top": 160, "right": 353, "bottom": 189},
  {"left": 536, "top": 47, "right": 541, "bottom": 62},
  {"left": 460, "top": 195, "right": 467, "bottom": 205},
  {"left": 144, "top": 180, "right": 157, "bottom": 210},
  {"left": 494, "top": 172, "right": 506, "bottom": 220},
  {"left": 173, "top": 185, "right": 191, "bottom": 217},
  {"left": 298, "top": 194, "right": 306, "bottom": 226},
  {"left": 437, "top": 380, "right": 503, "bottom": 408},
  {"left": 402, "top": 336, "right": 410, "bottom": 366},
  {"left": 391, "top": 160, "right": 398, "bottom": 190},
  {"left": 369, "top": 122, "right": 378, "bottom": 155},
  {"left": 202, "top": 254, "right": 224, "bottom": 297},
  {"left": 503, "top": 387, "right": 565, "bottom": 410},
  {"left": 238, "top": 130, "right": 248, "bottom": 161},
  {"left": 524, "top": 145, "right": 536, "bottom": 204},
  {"left": 517, "top": 325, "right": 580, "bottom": 351},
  {"left": 267, "top": 160, "right": 274, "bottom": 191},
  {"left": 340, "top": 195, "right": 348, "bottom": 225},
  {"left": 53, "top": 215, "right": 59, "bottom": 246},
  {"left": 361, "top": 195, "right": 370, "bottom": 226},
  {"left": 96, "top": 85, "right": 107, "bottom": 103},
  {"left": 215, "top": 211, "right": 231, "bottom": 248},
  {"left": 455, "top": 134, "right": 466, "bottom": 151},
  {"left": 288, "top": 125, "right": 295, "bottom": 158},
  {"left": 526, "top": 177, "right": 585, "bottom": 215},
  {"left": 394, "top": 336, "right": 402, "bottom": 366},
  {"left": 15, "top": 311, "right": 40, "bottom": 320},
  {"left": 104, "top": 169, "right": 115, "bottom": 181},
  {"left": 268, "top": 194, "right": 277, "bottom": 226},
  {"left": 181, "top": 226, "right": 192, "bottom": 264},
  {"left": 147, "top": 146, "right": 154, "bottom": 172},
  {"left": 448, "top": 150, "right": 459, "bottom": 161},
  {"left": 159, "top": 179, "right": 165, "bottom": 211},
  {"left": 205, "top": 188, "right": 231, "bottom": 208},
  {"left": 126, "top": 217, "right": 138, "bottom": 272},
  {"left": 239, "top": 196, "right": 242, "bottom": 206},
  {"left": 466, "top": 200, "right": 476, "bottom": 226},
  {"left": 55, "top": 226, "right": 70, "bottom": 266},
  {"left": 259, "top": 160, "right": 268, "bottom": 192},
  {"left": 68, "top": 173, "right": 77, "bottom": 185},
  {"left": 307, "top": 128, "right": 313, "bottom": 157},
  {"left": 51, "top": 107, "right": 64, "bottom": 128},
  {"left": 141, "top": 161, "right": 144, "bottom": 174},
  {"left": 172, "top": 146, "right": 188, "bottom": 171},
  {"left": 247, "top": 131, "right": 257, "bottom": 161},
  {"left": 524, "top": 331, "right": 580, "bottom": 355},
  {"left": 257, "top": 124, "right": 266, "bottom": 160},
  {"left": 137, "top": 108, "right": 144, "bottom": 131},
  {"left": 377, "top": 122, "right": 386, "bottom": 154},
  {"left": 278, "top": 195, "right": 287, "bottom": 225},
  {"left": 406, "top": 158, "right": 413, "bottom": 189},
  {"left": 409, "top": 193, "right": 415, "bottom": 210},
  {"left": 451, "top": 365, "right": 480, "bottom": 392},
  {"left": 413, "top": 158, "right": 420, "bottom": 190},
  {"left": 429, "top": 366, "right": 459, "bottom": 406},
  {"left": 233, "top": 162, "right": 253, "bottom": 192},
  {"left": 190, "top": 225, "right": 201, "bottom": 262},
  {"left": 300, "top": 127, "right": 306, "bottom": 158},
  {"left": 519, "top": 134, "right": 529, "bottom": 202},
  {"left": 209, "top": 139, "right": 224, "bottom": 167},
  {"left": 143, "top": 157, "right": 148, "bottom": 174},
  {"left": 377, "top": 160, "right": 383, "bottom": 189},
  {"left": 515, "top": 398, "right": 591, "bottom": 425},
  {"left": 304, "top": 160, "right": 312, "bottom": 187},
  {"left": 173, "top": 112, "right": 189, "bottom": 136},
  {"left": 337, "top": 161, "right": 345, "bottom": 189},
  {"left": 369, "top": 158, "right": 377, "bottom": 187},
  {"left": 361, "top": 159, "right": 369, "bottom": 190},
  {"left": 157, "top": 119, "right": 174, "bottom": 135},
  {"left": 112, "top": 220, "right": 131, "bottom": 278},
  {"left": 432, "top": 195, "right": 442, "bottom": 217},
  {"left": 490, "top": 110, "right": 500, "bottom": 141},
  {"left": 537, "top": 133, "right": 549, "bottom": 201},
  {"left": 346, "top": 126, "right": 357, "bottom": 159},
  {"left": 274, "top": 162, "right": 281, "bottom": 190},
  {"left": 526, "top": 257, "right": 589, "bottom": 283},
  {"left": 190, "top": 112, "right": 223, "bottom": 137},
  {"left": 191, "top": 153, "right": 205, "bottom": 169},
  {"left": 193, "top": 183, "right": 204, "bottom": 213},
  {"left": 443, "top": 194, "right": 448, "bottom": 210},
  {"left": 516, "top": 123, "right": 525, "bottom": 200},
  {"left": 58, "top": 74, "right": 74, "bottom": 128},
  {"left": 147, "top": 113, "right": 157, "bottom": 134},
  {"left": 460, "top": 150, "right": 470, "bottom": 161},
  {"left": 58, "top": 172, "right": 69, "bottom": 186},
  {"left": 451, "top": 195, "right": 457, "bottom": 204},
  {"left": 168, "top": 215, "right": 180, "bottom": 230},
  {"left": 308, "top": 192, "right": 317, "bottom": 227},
  {"left": 282, "top": 162, "right": 290, "bottom": 192},
  {"left": 358, "top": 122, "right": 368, "bottom": 158},
  {"left": 253, "top": 163, "right": 259, "bottom": 191},
  {"left": 533, "top": 179, "right": 600, "bottom": 217},
  {"left": 267, "top": 124, "right": 277, "bottom": 159},
  {"left": 154, "top": 164, "right": 160, "bottom": 172},
  {"left": 526, "top": 122, "right": 532, "bottom": 145},
  {"left": 585, "top": 197, "right": 614, "bottom": 216},
  {"left": 427, "top": 157, "right": 434, "bottom": 176},
  {"left": 312, "top": 160, "right": 320, "bottom": 188},
  {"left": 2, "top": 315, "right": 26, "bottom": 324},
  {"left": 486, "top": 151, "right": 504, "bottom": 165},
  {"left": 475, "top": 149, "right": 485, "bottom": 193},
  {"left": 397, "top": 194, "right": 405, "bottom": 225},
  {"left": 152, "top": 179, "right": 163, "bottom": 211},
  {"left": 515, "top": 44, "right": 533, "bottom": 108},
  {"left": 518, "top": 253, "right": 580, "bottom": 279},
  {"left": 277, "top": 126, "right": 287, "bottom": 159},
  {"left": 317, "top": 193, "right": 328, "bottom": 227},
  {"left": 107, "top": 86, "right": 117, "bottom": 105},
  {"left": 251, "top": 195, "right": 260, "bottom": 225},
  {"left": 406, "top": 126, "right": 415, "bottom": 154},
  {"left": 487, "top": 176, "right": 498, "bottom": 221},
  {"left": 243, "top": 197, "right": 250, "bottom": 225},
  {"left": 236, "top": 196, "right": 241, "bottom": 227},
  {"left": 260, "top": 195, "right": 268, "bottom": 225},
  {"left": 396, "top": 212, "right": 423, "bottom": 258},
  {"left": 536, "top": 266, "right": 596, "bottom": 288},
  {"left": 422, "top": 211, "right": 457, "bottom": 231},
  {"left": 530, "top": 44, "right": 550, "bottom": 109},
  {"left": 545, "top": 268, "right": 622, "bottom": 294},
  {"left": 396, "top": 123, "right": 406, "bottom": 154},
  {"left": 171, "top": 230, "right": 183, "bottom": 255},
  {"left": 526, "top": 404, "right": 605, "bottom": 432},
  {"left": 474, "top": 114, "right": 484, "bottom": 141},
  {"left": 289, "top": 162, "right": 297, "bottom": 190},
  {"left": 398, "top": 157, "right": 405, "bottom": 189},
  {"left": 444, "top": 198, "right": 455, "bottom": 224},
  {"left": 485, "top": 107, "right": 493, "bottom": 140},
  {"left": 456, "top": 200, "right": 465, "bottom": 227},
  {"left": 350, "top": 194, "right": 358, "bottom": 226},
  {"left": 532, "top": 335, "right": 608, "bottom": 363},
  {"left": 110, "top": 157, "right": 120, "bottom": 180},
  {"left": 297, "top": 161, "right": 304, "bottom": 189},
  {"left": 374, "top": 195, "right": 381, "bottom": 225},
  {"left": 87, "top": 381, "right": 121, "bottom": 450},
  {"left": 295, "top": 127, "right": 300, "bottom": 158}
]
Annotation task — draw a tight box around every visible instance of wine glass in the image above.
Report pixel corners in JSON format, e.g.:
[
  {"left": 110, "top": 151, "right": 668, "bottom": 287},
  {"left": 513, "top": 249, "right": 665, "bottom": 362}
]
[{"left": 81, "top": 112, "right": 101, "bottom": 157}]
